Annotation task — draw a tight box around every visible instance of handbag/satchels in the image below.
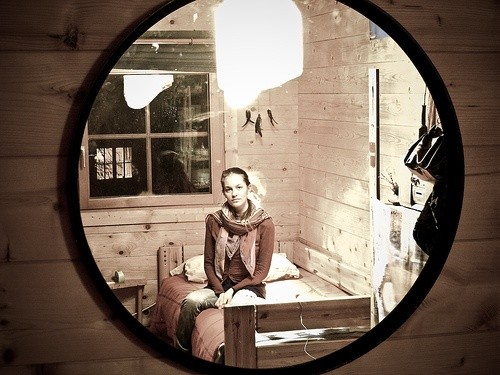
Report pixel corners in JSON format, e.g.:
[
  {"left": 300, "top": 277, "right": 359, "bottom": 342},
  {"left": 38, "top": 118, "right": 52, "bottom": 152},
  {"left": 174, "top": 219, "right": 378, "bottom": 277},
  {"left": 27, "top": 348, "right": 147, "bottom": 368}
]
[{"left": 403, "top": 124, "right": 445, "bottom": 182}]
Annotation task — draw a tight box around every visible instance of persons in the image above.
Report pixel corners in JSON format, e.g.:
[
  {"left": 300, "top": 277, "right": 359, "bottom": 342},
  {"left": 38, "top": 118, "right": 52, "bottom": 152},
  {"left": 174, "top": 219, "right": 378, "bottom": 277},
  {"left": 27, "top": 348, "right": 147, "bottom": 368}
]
[{"left": 175, "top": 167, "right": 274, "bottom": 355}]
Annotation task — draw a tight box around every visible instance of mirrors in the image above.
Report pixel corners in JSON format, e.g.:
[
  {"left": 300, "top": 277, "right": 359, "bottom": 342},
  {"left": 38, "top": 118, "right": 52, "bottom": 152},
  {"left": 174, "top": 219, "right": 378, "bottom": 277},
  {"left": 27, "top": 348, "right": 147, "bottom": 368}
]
[{"left": 56, "top": 2, "right": 464, "bottom": 374}]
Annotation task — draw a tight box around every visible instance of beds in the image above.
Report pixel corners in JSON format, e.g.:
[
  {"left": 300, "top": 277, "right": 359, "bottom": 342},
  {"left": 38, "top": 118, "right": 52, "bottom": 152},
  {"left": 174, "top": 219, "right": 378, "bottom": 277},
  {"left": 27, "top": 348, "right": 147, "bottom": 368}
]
[{"left": 148, "top": 241, "right": 370, "bottom": 369}]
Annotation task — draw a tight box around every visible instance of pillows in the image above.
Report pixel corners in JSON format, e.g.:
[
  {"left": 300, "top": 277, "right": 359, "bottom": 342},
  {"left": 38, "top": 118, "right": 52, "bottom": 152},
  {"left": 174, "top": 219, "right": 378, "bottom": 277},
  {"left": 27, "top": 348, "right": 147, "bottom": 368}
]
[{"left": 169, "top": 253, "right": 299, "bottom": 282}]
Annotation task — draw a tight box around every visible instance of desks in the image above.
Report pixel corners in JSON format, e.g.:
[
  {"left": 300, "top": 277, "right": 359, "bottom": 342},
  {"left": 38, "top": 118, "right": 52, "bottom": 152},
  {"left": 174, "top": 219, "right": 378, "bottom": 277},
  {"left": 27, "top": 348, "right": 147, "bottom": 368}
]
[{"left": 106, "top": 279, "right": 146, "bottom": 324}]
